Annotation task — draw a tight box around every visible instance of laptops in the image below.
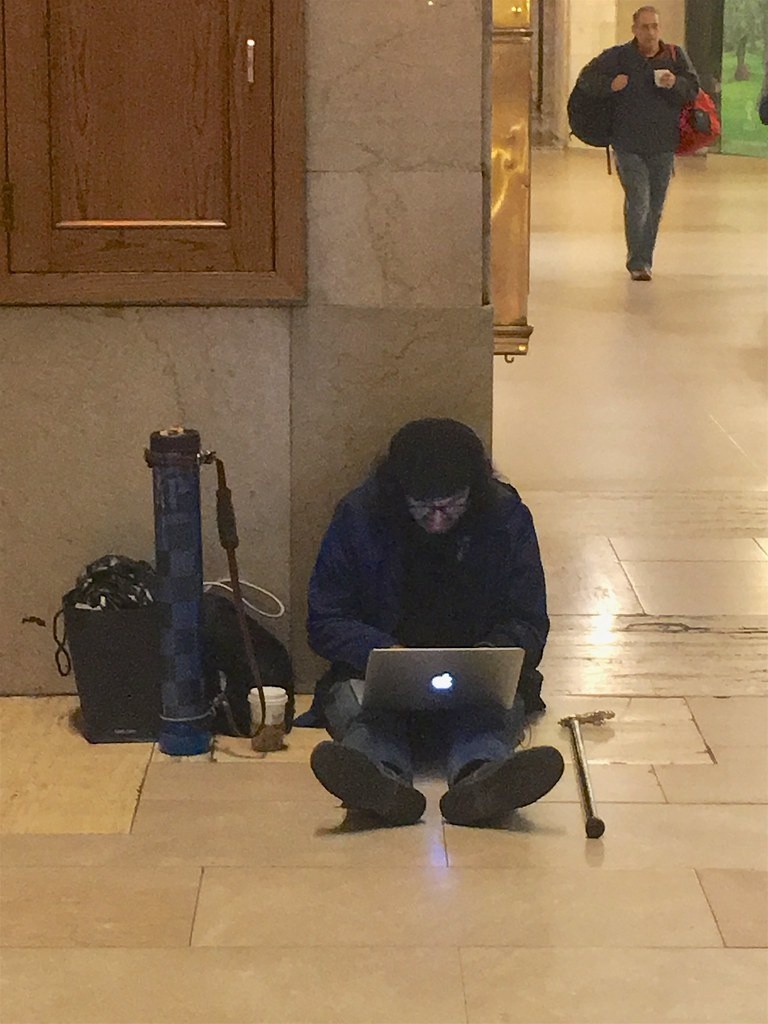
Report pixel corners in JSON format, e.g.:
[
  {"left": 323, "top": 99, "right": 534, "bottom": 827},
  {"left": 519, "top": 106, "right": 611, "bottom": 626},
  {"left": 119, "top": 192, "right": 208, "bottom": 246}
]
[{"left": 348, "top": 649, "right": 524, "bottom": 710}]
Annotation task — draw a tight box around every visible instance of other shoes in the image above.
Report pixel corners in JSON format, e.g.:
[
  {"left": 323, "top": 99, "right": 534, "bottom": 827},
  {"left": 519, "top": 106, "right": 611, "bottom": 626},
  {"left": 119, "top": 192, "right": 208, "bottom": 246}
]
[
  {"left": 309, "top": 742, "right": 426, "bottom": 822},
  {"left": 629, "top": 266, "right": 651, "bottom": 283},
  {"left": 439, "top": 744, "right": 564, "bottom": 826}
]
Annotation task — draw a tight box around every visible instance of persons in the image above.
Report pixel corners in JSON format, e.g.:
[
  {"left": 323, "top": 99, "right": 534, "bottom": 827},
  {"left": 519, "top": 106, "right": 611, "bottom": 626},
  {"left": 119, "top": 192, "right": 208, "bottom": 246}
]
[
  {"left": 289, "top": 415, "right": 564, "bottom": 824},
  {"left": 601, "top": 6, "right": 702, "bottom": 280}
]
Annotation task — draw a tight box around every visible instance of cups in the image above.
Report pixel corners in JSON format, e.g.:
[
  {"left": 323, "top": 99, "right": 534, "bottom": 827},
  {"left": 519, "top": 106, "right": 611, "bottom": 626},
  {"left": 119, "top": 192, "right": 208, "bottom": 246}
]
[
  {"left": 247, "top": 685, "right": 290, "bottom": 752},
  {"left": 654, "top": 68, "right": 669, "bottom": 88}
]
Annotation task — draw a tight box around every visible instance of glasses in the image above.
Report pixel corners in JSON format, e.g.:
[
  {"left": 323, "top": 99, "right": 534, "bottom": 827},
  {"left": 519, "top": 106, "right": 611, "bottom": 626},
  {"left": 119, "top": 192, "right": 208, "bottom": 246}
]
[{"left": 404, "top": 495, "right": 469, "bottom": 518}]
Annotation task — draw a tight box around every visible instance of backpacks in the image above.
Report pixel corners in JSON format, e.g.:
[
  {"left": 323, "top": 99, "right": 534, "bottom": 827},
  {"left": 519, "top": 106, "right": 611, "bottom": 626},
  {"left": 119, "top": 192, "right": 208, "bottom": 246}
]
[
  {"left": 569, "top": 45, "right": 622, "bottom": 147},
  {"left": 668, "top": 44, "right": 720, "bottom": 155}
]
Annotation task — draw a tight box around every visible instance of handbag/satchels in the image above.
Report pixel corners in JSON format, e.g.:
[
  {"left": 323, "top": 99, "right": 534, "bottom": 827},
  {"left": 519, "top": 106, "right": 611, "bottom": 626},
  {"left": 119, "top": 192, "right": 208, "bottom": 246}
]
[
  {"left": 51, "top": 586, "right": 167, "bottom": 745},
  {"left": 202, "top": 596, "right": 296, "bottom": 739}
]
